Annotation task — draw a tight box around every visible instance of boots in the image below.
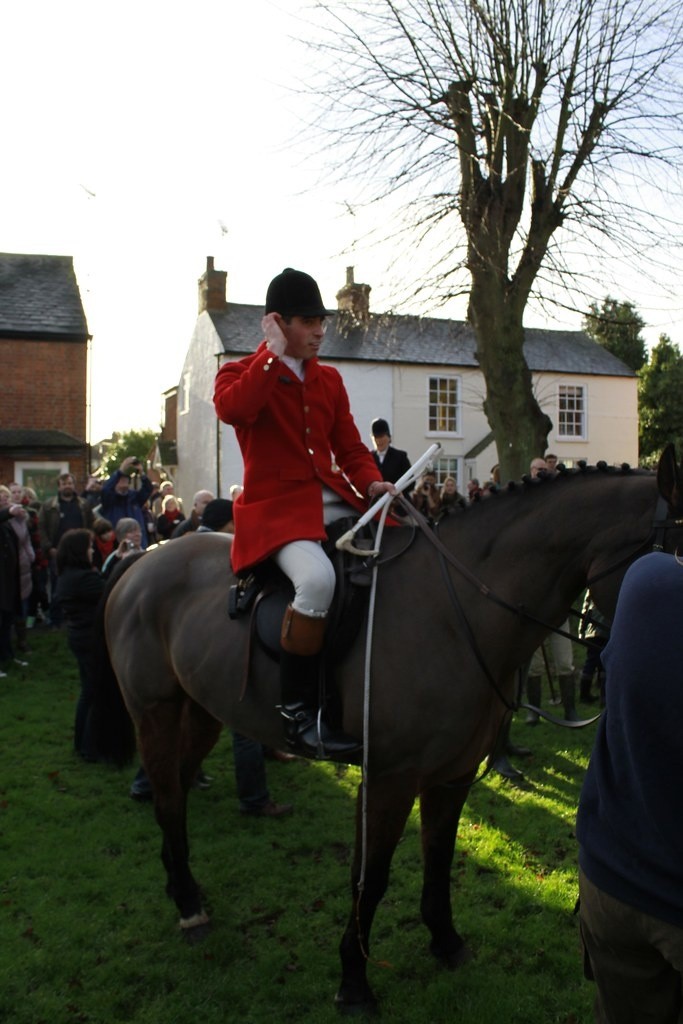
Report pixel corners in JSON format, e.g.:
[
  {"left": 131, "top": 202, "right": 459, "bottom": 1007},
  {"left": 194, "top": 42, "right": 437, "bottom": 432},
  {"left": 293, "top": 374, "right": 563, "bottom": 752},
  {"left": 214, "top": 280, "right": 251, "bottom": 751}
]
[
  {"left": 526, "top": 675, "right": 542, "bottom": 727},
  {"left": 559, "top": 673, "right": 584, "bottom": 728},
  {"left": 15, "top": 620, "right": 30, "bottom": 651},
  {"left": 579, "top": 671, "right": 600, "bottom": 705},
  {"left": 276, "top": 602, "right": 363, "bottom": 755}
]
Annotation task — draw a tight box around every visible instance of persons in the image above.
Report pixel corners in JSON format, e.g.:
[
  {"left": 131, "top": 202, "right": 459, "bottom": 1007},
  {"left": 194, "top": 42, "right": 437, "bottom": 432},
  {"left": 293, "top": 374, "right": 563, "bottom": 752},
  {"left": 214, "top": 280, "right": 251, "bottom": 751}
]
[
  {"left": 371, "top": 419, "right": 612, "bottom": 781},
  {"left": 0, "top": 455, "right": 294, "bottom": 819},
  {"left": 213, "top": 269, "right": 398, "bottom": 760},
  {"left": 570, "top": 551, "right": 683, "bottom": 1024}
]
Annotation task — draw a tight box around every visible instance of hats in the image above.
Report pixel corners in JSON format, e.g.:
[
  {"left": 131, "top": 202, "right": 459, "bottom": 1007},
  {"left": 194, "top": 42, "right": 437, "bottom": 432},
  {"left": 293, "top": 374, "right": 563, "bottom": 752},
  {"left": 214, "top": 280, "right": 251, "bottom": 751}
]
[
  {"left": 372, "top": 418, "right": 390, "bottom": 438},
  {"left": 265, "top": 268, "right": 336, "bottom": 318}
]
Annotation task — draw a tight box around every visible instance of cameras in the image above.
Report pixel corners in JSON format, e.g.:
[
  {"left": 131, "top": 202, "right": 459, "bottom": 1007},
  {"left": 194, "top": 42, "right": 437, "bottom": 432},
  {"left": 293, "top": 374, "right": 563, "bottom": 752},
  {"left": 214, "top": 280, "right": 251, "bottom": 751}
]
[
  {"left": 126, "top": 542, "right": 135, "bottom": 550},
  {"left": 132, "top": 459, "right": 141, "bottom": 466},
  {"left": 423, "top": 481, "right": 430, "bottom": 491}
]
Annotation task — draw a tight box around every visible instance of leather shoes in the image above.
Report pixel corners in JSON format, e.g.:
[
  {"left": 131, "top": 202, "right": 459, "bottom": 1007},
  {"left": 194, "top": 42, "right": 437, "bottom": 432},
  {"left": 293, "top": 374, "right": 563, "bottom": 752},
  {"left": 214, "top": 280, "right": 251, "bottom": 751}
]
[{"left": 238, "top": 800, "right": 292, "bottom": 818}]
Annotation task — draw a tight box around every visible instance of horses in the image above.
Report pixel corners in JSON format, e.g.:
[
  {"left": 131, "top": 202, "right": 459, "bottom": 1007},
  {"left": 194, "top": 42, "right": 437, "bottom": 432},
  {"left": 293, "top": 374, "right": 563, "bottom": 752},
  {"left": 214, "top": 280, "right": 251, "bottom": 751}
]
[{"left": 97, "top": 440, "right": 683, "bottom": 1024}]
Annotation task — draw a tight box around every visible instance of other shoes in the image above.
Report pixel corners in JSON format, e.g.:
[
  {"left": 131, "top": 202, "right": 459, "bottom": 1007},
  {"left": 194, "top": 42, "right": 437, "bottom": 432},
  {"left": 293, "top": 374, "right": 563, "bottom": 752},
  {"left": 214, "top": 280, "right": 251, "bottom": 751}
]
[
  {"left": 47, "top": 622, "right": 63, "bottom": 631},
  {"left": 266, "top": 747, "right": 297, "bottom": 761},
  {"left": 488, "top": 749, "right": 524, "bottom": 783},
  {"left": 25, "top": 617, "right": 35, "bottom": 628},
  {"left": 503, "top": 740, "right": 530, "bottom": 757},
  {"left": 0, "top": 670, "right": 7, "bottom": 678},
  {"left": 130, "top": 766, "right": 150, "bottom": 804},
  {"left": 13, "top": 657, "right": 28, "bottom": 666}
]
